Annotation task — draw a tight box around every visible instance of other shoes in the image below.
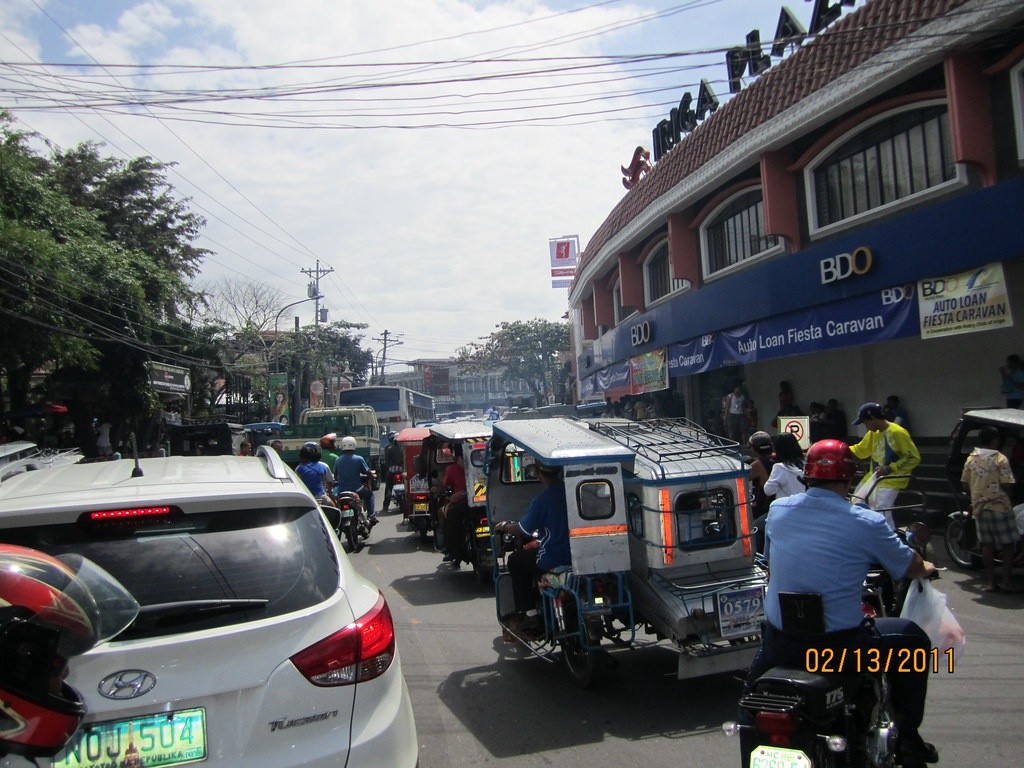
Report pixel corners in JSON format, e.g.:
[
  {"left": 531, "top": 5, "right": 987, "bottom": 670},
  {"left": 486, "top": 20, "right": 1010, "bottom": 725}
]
[
  {"left": 383, "top": 505, "right": 388, "bottom": 510},
  {"left": 515, "top": 613, "right": 543, "bottom": 630},
  {"left": 443, "top": 553, "right": 457, "bottom": 561},
  {"left": 895, "top": 735, "right": 939, "bottom": 764},
  {"left": 999, "top": 584, "right": 1024, "bottom": 593},
  {"left": 984, "top": 583, "right": 997, "bottom": 591}
]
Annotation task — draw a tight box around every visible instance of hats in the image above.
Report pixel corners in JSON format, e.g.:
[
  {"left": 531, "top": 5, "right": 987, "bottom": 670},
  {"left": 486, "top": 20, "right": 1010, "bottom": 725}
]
[
  {"left": 852, "top": 403, "right": 882, "bottom": 426},
  {"left": 748, "top": 431, "right": 772, "bottom": 450}
]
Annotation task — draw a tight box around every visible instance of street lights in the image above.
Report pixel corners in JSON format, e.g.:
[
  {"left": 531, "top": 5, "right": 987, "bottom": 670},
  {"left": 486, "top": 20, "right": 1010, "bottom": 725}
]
[
  {"left": 375, "top": 341, "right": 404, "bottom": 386},
  {"left": 276, "top": 294, "right": 326, "bottom": 374}
]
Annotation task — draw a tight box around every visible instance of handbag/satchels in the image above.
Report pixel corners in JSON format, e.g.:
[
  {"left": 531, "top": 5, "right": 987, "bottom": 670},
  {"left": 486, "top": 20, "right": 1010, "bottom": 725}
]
[{"left": 900, "top": 577, "right": 966, "bottom": 653}]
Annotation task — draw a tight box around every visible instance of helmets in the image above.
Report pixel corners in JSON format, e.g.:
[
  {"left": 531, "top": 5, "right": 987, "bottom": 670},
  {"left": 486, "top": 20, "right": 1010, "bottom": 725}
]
[
  {"left": 388, "top": 431, "right": 399, "bottom": 443},
  {"left": 300, "top": 441, "right": 321, "bottom": 463},
  {"left": 804, "top": 439, "right": 857, "bottom": 480},
  {"left": 341, "top": 436, "right": 357, "bottom": 450},
  {"left": 320, "top": 433, "right": 336, "bottom": 449},
  {"left": 0, "top": 544, "right": 98, "bottom": 757}
]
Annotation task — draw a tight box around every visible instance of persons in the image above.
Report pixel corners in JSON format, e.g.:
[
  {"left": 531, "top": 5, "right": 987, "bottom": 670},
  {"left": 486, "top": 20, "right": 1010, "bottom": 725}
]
[
  {"left": 319, "top": 433, "right": 339, "bottom": 473},
  {"left": 0, "top": 543, "right": 141, "bottom": 768},
  {"left": 809, "top": 398, "right": 847, "bottom": 440},
  {"left": 96, "top": 417, "right": 114, "bottom": 456},
  {"left": 495, "top": 458, "right": 573, "bottom": 630},
  {"left": 999, "top": 354, "right": 1024, "bottom": 409},
  {"left": 240, "top": 442, "right": 252, "bottom": 456},
  {"left": 334, "top": 436, "right": 379, "bottom": 523},
  {"left": 772, "top": 380, "right": 794, "bottom": 428},
  {"left": 747, "top": 431, "right": 776, "bottom": 516},
  {"left": 602, "top": 394, "right": 649, "bottom": 421},
  {"left": 763, "top": 439, "right": 939, "bottom": 763},
  {"left": 439, "top": 443, "right": 467, "bottom": 562},
  {"left": 754, "top": 432, "right": 806, "bottom": 544},
  {"left": 882, "top": 396, "right": 912, "bottom": 434},
  {"left": 295, "top": 442, "right": 336, "bottom": 507},
  {"left": 720, "top": 383, "right": 758, "bottom": 444},
  {"left": 383, "top": 431, "right": 404, "bottom": 512},
  {"left": 961, "top": 426, "right": 1024, "bottom": 594},
  {"left": 848, "top": 402, "right": 922, "bottom": 529}
]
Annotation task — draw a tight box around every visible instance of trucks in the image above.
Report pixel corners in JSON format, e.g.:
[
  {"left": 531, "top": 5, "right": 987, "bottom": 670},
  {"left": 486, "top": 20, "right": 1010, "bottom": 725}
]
[{"left": 266, "top": 403, "right": 388, "bottom": 491}]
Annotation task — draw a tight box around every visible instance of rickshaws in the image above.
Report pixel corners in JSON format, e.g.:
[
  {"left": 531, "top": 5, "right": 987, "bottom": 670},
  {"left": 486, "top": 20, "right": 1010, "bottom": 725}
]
[
  {"left": 943, "top": 405, "right": 1024, "bottom": 596},
  {"left": 469, "top": 413, "right": 773, "bottom": 693},
  {"left": 416, "top": 420, "right": 535, "bottom": 592},
  {"left": 697, "top": 451, "right": 936, "bottom": 565},
  {"left": 394, "top": 427, "right": 453, "bottom": 546}
]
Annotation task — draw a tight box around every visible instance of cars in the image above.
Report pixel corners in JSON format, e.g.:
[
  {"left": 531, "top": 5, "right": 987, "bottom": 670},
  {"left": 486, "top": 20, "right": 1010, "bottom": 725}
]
[{"left": 411, "top": 402, "right": 611, "bottom": 421}]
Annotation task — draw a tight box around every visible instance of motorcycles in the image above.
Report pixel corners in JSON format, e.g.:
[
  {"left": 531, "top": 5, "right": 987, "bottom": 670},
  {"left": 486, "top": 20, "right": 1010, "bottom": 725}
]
[{"left": 723, "top": 521, "right": 950, "bottom": 768}]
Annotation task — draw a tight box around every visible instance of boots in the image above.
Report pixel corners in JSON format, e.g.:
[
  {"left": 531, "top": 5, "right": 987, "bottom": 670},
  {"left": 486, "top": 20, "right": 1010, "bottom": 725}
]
[{"left": 370, "top": 514, "right": 378, "bottom": 524}]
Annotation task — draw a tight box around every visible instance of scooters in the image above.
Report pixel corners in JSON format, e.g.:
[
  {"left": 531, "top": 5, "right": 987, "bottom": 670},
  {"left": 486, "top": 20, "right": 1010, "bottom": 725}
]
[
  {"left": 378, "top": 458, "right": 407, "bottom": 514},
  {"left": 336, "top": 469, "right": 378, "bottom": 552}
]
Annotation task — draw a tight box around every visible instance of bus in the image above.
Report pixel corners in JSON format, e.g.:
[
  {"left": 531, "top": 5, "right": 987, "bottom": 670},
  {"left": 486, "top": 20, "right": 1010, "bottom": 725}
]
[{"left": 335, "top": 384, "right": 439, "bottom": 459}]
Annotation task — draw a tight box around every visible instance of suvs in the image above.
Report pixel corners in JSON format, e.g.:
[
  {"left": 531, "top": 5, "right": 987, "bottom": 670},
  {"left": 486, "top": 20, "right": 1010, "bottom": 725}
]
[{"left": 0, "top": 439, "right": 421, "bottom": 768}]
